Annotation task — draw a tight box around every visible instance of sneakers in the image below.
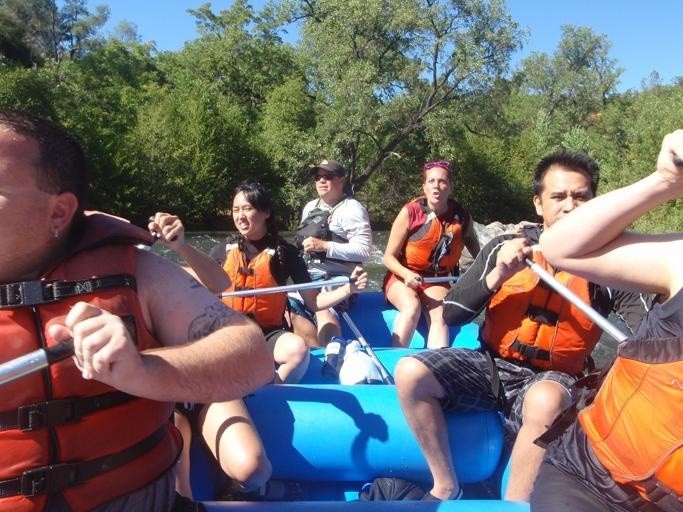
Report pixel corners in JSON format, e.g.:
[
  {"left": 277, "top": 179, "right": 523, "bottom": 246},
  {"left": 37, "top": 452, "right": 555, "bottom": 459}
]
[
  {"left": 420, "top": 486, "right": 463, "bottom": 500},
  {"left": 223, "top": 478, "right": 304, "bottom": 501}
]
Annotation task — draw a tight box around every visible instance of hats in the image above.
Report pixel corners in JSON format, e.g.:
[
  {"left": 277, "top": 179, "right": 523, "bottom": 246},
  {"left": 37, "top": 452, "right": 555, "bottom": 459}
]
[{"left": 310, "top": 160, "right": 345, "bottom": 179}]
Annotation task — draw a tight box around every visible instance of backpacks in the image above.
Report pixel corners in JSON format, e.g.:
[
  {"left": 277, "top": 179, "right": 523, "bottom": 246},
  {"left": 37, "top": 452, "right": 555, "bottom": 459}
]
[{"left": 296, "top": 209, "right": 330, "bottom": 258}]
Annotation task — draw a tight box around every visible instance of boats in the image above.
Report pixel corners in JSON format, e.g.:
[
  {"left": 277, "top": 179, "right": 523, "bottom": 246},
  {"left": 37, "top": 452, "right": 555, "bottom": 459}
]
[{"left": 189, "top": 291, "right": 532, "bottom": 512}]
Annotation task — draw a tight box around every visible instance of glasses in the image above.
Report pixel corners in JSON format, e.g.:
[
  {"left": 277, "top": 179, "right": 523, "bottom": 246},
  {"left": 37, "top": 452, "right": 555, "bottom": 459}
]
[
  {"left": 424, "top": 161, "right": 449, "bottom": 168},
  {"left": 314, "top": 174, "right": 333, "bottom": 180}
]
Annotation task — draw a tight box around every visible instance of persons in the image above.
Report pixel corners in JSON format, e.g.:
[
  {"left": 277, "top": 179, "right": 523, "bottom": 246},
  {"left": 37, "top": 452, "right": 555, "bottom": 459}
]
[
  {"left": 380, "top": 164, "right": 482, "bottom": 350},
  {"left": 281, "top": 159, "right": 374, "bottom": 351},
  {"left": 1, "top": 104, "right": 276, "bottom": 512},
  {"left": 528, "top": 127, "right": 682, "bottom": 511},
  {"left": 142, "top": 208, "right": 274, "bottom": 502},
  {"left": 392, "top": 150, "right": 657, "bottom": 501},
  {"left": 204, "top": 180, "right": 367, "bottom": 385}
]
